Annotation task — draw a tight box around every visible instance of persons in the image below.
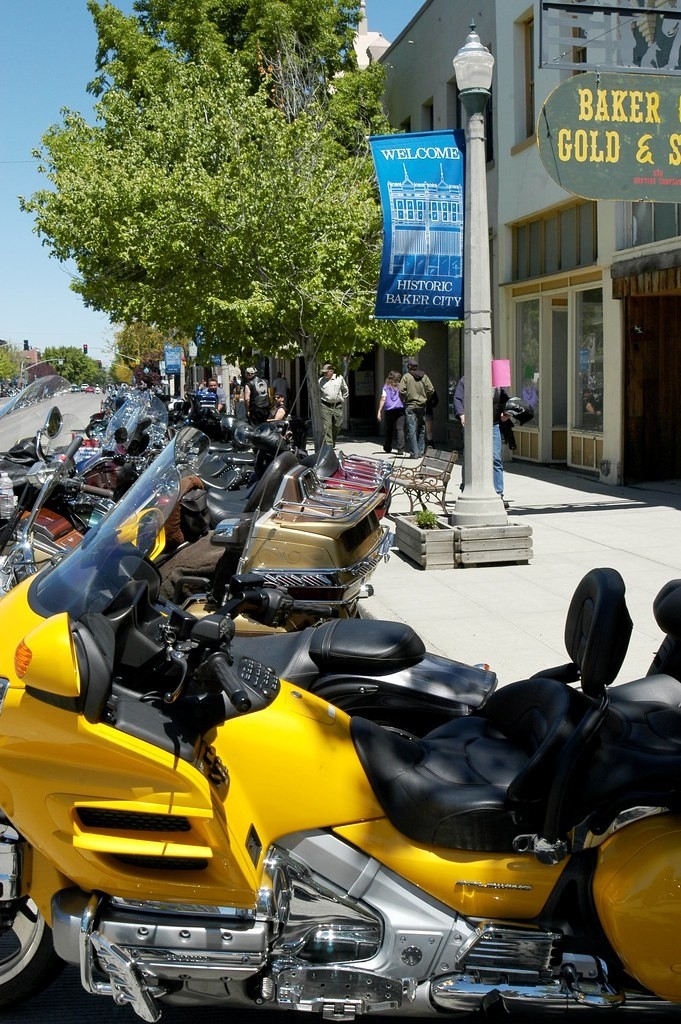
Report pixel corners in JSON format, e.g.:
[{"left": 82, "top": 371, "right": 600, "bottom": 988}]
[
  {"left": 398, "top": 360, "right": 435, "bottom": 459},
  {"left": 453, "top": 353, "right": 516, "bottom": 506},
  {"left": 316, "top": 364, "right": 349, "bottom": 448},
  {"left": 375, "top": 370, "right": 409, "bottom": 454},
  {"left": 420, "top": 389, "right": 439, "bottom": 448},
  {"left": 179, "top": 365, "right": 299, "bottom": 429}
]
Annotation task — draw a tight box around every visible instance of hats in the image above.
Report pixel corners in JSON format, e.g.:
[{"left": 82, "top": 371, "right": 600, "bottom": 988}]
[
  {"left": 245, "top": 367, "right": 258, "bottom": 374},
  {"left": 404, "top": 360, "right": 418, "bottom": 367},
  {"left": 320, "top": 364, "right": 334, "bottom": 374}
]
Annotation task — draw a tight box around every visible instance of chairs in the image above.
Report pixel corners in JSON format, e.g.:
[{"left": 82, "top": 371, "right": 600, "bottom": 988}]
[
  {"left": 353, "top": 568, "right": 632, "bottom": 851},
  {"left": 169, "top": 451, "right": 298, "bottom": 599},
  {"left": 206, "top": 445, "right": 338, "bottom": 529},
  {"left": 612, "top": 579, "right": 681, "bottom": 801}
]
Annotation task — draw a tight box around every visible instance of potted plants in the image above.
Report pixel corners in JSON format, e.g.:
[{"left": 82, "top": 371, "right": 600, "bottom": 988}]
[{"left": 395, "top": 510, "right": 456, "bottom": 571}]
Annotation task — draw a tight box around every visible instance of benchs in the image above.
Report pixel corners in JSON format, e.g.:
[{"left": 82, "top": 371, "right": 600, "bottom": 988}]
[{"left": 390, "top": 448, "right": 457, "bottom": 514}]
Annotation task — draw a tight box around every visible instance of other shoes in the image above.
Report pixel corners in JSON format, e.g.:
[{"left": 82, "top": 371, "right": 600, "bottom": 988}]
[
  {"left": 409, "top": 452, "right": 423, "bottom": 459},
  {"left": 426, "top": 440, "right": 438, "bottom": 452},
  {"left": 398, "top": 450, "right": 405, "bottom": 454},
  {"left": 384, "top": 447, "right": 392, "bottom": 453}
]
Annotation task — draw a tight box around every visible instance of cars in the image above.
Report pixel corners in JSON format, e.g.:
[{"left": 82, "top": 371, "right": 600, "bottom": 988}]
[{"left": 69, "top": 382, "right": 130, "bottom": 395}]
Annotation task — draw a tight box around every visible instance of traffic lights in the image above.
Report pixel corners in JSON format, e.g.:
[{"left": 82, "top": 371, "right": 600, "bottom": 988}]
[
  {"left": 83, "top": 344, "right": 88, "bottom": 354},
  {"left": 98, "top": 360, "right": 102, "bottom": 370}
]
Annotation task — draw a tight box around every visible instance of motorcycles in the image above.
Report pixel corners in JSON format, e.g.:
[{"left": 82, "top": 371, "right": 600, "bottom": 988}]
[
  {"left": 0, "top": 422, "right": 680, "bottom": 1022},
  {"left": 0, "top": 387, "right": 500, "bottom": 746}
]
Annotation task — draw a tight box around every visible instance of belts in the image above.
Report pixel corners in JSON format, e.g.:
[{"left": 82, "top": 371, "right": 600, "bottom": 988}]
[
  {"left": 320, "top": 399, "right": 342, "bottom": 409},
  {"left": 274, "top": 396, "right": 281, "bottom": 399}
]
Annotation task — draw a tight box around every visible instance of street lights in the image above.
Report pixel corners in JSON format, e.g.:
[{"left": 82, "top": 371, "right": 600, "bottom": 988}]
[{"left": 442, "top": 16, "right": 510, "bottom": 526}]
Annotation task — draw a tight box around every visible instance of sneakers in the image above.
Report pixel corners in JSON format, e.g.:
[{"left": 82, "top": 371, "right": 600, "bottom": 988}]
[{"left": 498, "top": 495, "right": 509, "bottom": 508}]
